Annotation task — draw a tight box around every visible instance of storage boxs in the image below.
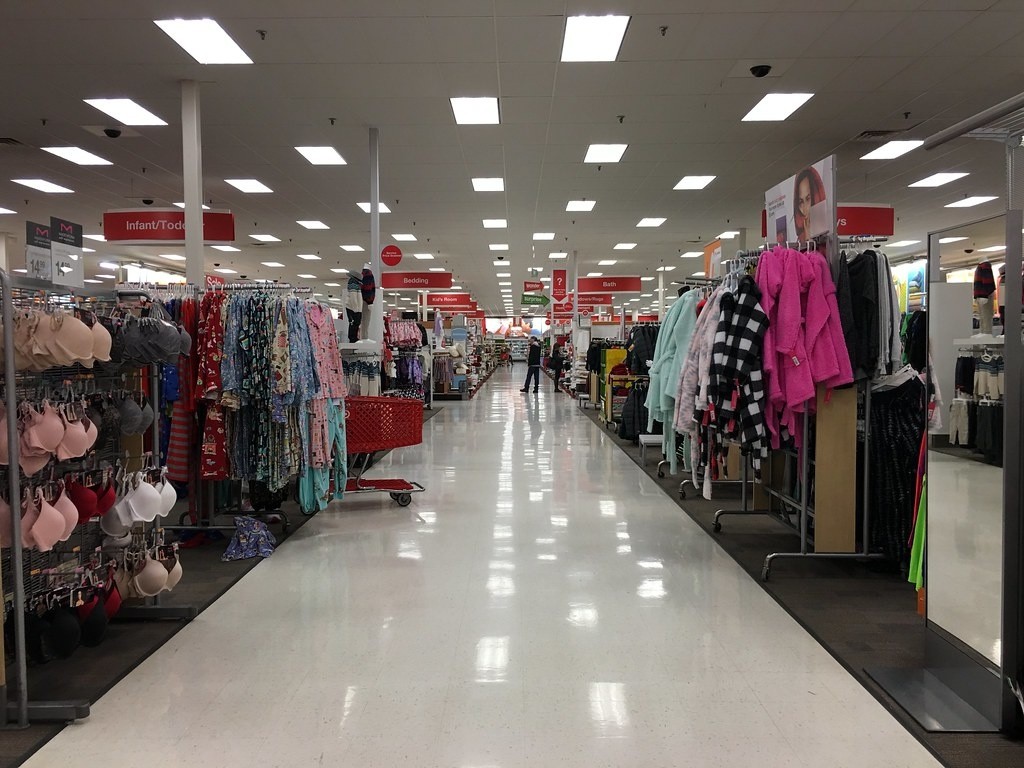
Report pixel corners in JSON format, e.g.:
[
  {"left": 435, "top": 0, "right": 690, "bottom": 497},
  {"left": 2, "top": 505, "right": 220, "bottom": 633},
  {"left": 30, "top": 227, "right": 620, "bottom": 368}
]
[{"left": 713, "top": 443, "right": 742, "bottom": 481}]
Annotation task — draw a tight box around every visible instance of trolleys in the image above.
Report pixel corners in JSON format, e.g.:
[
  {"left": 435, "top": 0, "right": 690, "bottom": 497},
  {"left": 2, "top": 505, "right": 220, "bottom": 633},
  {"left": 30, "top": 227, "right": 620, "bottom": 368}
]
[
  {"left": 294, "top": 395, "right": 426, "bottom": 516},
  {"left": 500, "top": 353, "right": 510, "bottom": 367}
]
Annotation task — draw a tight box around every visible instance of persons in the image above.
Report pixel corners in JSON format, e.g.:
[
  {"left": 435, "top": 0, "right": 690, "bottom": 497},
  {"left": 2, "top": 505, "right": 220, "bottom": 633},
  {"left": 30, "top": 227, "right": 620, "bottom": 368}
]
[
  {"left": 519, "top": 336, "right": 541, "bottom": 393},
  {"left": 971, "top": 262, "right": 996, "bottom": 338},
  {"left": 552, "top": 343, "right": 565, "bottom": 393},
  {"left": 793, "top": 166, "right": 827, "bottom": 258},
  {"left": 360, "top": 262, "right": 377, "bottom": 344},
  {"left": 996, "top": 264, "right": 1005, "bottom": 338},
  {"left": 345, "top": 271, "right": 365, "bottom": 344},
  {"left": 434, "top": 311, "right": 446, "bottom": 350},
  {"left": 507, "top": 344, "right": 514, "bottom": 366}
]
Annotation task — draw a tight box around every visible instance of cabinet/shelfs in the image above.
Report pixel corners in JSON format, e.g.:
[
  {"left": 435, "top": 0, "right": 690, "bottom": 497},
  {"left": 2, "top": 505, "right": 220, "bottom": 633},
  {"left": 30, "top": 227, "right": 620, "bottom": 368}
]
[
  {"left": 753, "top": 383, "right": 858, "bottom": 553},
  {"left": 429, "top": 339, "right": 531, "bottom": 406},
  {"left": 539, "top": 332, "right": 632, "bottom": 399}
]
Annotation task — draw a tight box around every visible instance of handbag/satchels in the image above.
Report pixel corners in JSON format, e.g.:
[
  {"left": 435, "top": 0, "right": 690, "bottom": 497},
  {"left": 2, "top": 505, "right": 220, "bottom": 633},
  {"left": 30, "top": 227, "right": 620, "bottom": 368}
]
[{"left": 546, "top": 357, "right": 556, "bottom": 370}]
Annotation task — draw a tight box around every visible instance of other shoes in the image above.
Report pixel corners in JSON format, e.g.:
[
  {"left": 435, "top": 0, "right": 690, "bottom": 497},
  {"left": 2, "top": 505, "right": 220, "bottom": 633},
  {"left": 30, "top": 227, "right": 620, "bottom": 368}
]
[
  {"left": 554, "top": 389, "right": 562, "bottom": 393},
  {"left": 533, "top": 390, "right": 538, "bottom": 393},
  {"left": 520, "top": 388, "right": 529, "bottom": 393}
]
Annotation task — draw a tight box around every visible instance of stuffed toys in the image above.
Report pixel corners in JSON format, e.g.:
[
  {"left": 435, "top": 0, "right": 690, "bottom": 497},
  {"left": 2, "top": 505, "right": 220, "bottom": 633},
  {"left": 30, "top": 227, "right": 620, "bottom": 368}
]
[{"left": 516, "top": 321, "right": 531, "bottom": 338}]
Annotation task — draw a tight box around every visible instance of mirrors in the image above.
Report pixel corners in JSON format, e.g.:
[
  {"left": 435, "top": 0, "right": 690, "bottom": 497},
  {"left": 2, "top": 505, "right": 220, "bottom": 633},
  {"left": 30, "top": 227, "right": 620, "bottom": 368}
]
[{"left": 926, "top": 215, "right": 1006, "bottom": 468}]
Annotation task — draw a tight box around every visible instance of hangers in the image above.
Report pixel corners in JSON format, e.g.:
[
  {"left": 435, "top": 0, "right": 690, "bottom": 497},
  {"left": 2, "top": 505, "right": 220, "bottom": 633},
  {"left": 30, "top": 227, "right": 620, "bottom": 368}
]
[
  {"left": 866, "top": 363, "right": 920, "bottom": 396},
  {"left": 338, "top": 350, "right": 378, "bottom": 364},
  {"left": 959, "top": 347, "right": 999, "bottom": 359},
  {"left": 391, "top": 318, "right": 417, "bottom": 325},
  {"left": 634, "top": 382, "right": 648, "bottom": 390},
  {"left": 120, "top": 282, "right": 318, "bottom": 308},
  {"left": 633, "top": 320, "right": 661, "bottom": 328},
  {"left": 837, "top": 235, "right": 881, "bottom": 256},
  {"left": 951, "top": 391, "right": 1001, "bottom": 408},
  {"left": 690, "top": 239, "right": 819, "bottom": 302},
  {"left": 0, "top": 288, "right": 178, "bottom": 613}
]
